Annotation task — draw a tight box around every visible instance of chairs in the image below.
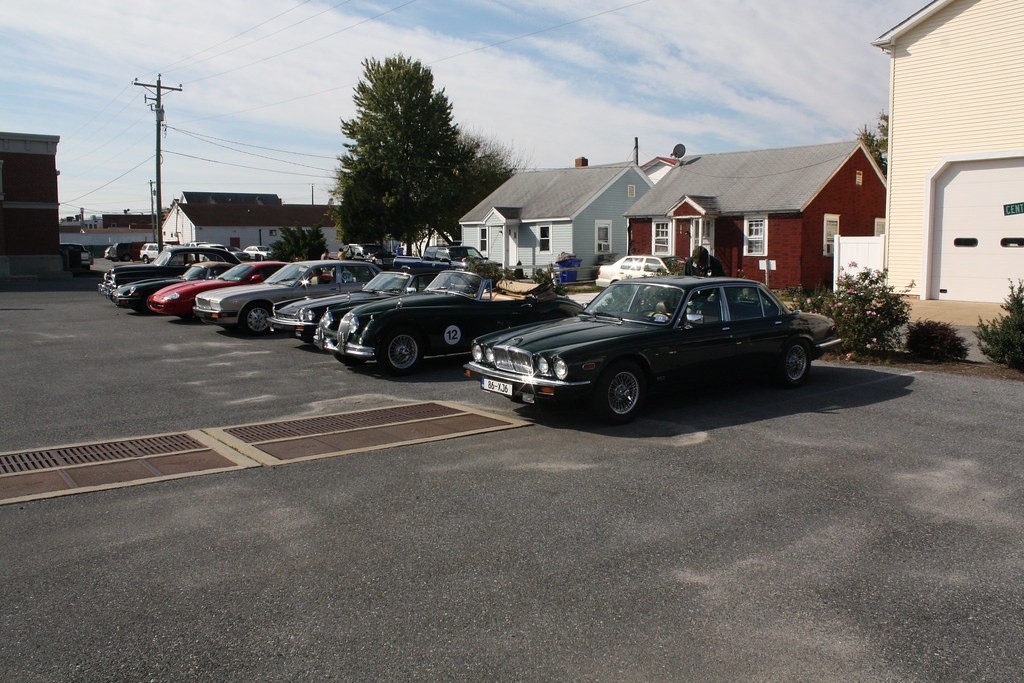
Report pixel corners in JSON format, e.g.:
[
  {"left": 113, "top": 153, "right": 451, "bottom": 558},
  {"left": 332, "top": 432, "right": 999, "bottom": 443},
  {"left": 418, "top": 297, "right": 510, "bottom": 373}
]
[
  {"left": 311, "top": 268, "right": 322, "bottom": 284},
  {"left": 692, "top": 296, "right": 705, "bottom": 314},
  {"left": 655, "top": 300, "right": 672, "bottom": 320},
  {"left": 330, "top": 267, "right": 344, "bottom": 283}
]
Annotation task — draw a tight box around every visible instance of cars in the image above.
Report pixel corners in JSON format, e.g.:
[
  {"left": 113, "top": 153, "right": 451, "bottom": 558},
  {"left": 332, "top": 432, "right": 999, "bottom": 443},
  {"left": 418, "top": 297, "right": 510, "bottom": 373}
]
[
  {"left": 312, "top": 269, "right": 587, "bottom": 376},
  {"left": 343, "top": 244, "right": 397, "bottom": 272},
  {"left": 243, "top": 245, "right": 271, "bottom": 262},
  {"left": 110, "top": 262, "right": 235, "bottom": 315},
  {"left": 197, "top": 243, "right": 227, "bottom": 263},
  {"left": 265, "top": 271, "right": 439, "bottom": 349},
  {"left": 104, "top": 241, "right": 145, "bottom": 262},
  {"left": 193, "top": 260, "right": 384, "bottom": 337},
  {"left": 462, "top": 275, "right": 843, "bottom": 425},
  {"left": 145, "top": 262, "right": 289, "bottom": 325},
  {"left": 594, "top": 254, "right": 687, "bottom": 287},
  {"left": 226, "top": 245, "right": 251, "bottom": 262},
  {"left": 139, "top": 243, "right": 158, "bottom": 264},
  {"left": 98, "top": 248, "right": 243, "bottom": 296}
]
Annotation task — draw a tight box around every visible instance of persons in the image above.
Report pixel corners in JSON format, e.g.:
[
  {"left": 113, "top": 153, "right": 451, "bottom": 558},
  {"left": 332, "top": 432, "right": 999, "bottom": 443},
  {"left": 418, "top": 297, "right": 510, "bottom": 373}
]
[
  {"left": 685, "top": 246, "right": 724, "bottom": 302},
  {"left": 338, "top": 246, "right": 350, "bottom": 260},
  {"left": 321, "top": 251, "right": 334, "bottom": 260}
]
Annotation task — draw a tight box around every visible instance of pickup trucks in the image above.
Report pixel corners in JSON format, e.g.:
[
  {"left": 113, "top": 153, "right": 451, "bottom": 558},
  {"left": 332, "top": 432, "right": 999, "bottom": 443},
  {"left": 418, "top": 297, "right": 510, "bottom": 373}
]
[{"left": 393, "top": 245, "right": 503, "bottom": 284}]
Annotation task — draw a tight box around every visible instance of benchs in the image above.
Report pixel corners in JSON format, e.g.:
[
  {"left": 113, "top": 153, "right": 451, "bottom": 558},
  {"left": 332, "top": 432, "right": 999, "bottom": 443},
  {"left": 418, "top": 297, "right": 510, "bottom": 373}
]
[
  {"left": 482, "top": 292, "right": 514, "bottom": 300},
  {"left": 705, "top": 301, "right": 765, "bottom": 320}
]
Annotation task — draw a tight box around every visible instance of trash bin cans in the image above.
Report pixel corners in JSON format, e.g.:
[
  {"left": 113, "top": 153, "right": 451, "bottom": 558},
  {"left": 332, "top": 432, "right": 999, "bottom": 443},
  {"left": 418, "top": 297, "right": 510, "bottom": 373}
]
[{"left": 555, "top": 259, "right": 583, "bottom": 282}]
